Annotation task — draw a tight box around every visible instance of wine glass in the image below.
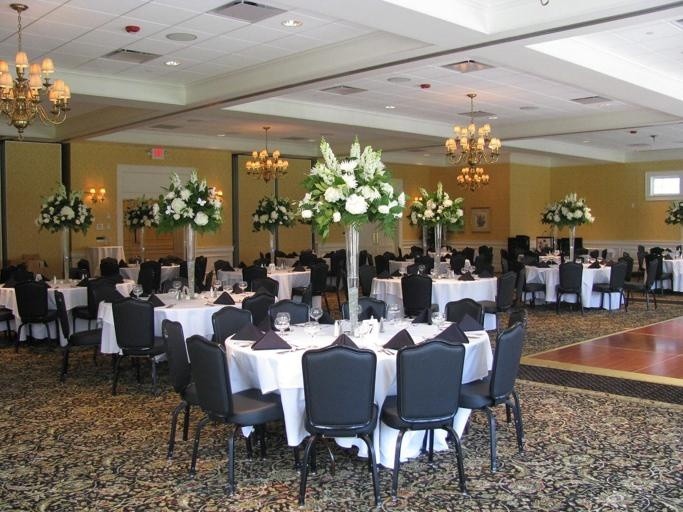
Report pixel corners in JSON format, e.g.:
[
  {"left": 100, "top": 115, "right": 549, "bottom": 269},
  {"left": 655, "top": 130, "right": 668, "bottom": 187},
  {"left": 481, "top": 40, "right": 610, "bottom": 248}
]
[
  {"left": 132, "top": 284, "right": 143, "bottom": 301},
  {"left": 238, "top": 281, "right": 247, "bottom": 297},
  {"left": 168, "top": 288, "right": 176, "bottom": 306},
  {"left": 429, "top": 311, "right": 446, "bottom": 335},
  {"left": 387, "top": 304, "right": 400, "bottom": 326},
  {"left": 460, "top": 265, "right": 475, "bottom": 276},
  {"left": 309, "top": 306, "right": 323, "bottom": 330},
  {"left": 172, "top": 281, "right": 182, "bottom": 297},
  {"left": 212, "top": 279, "right": 221, "bottom": 296},
  {"left": 272, "top": 311, "right": 290, "bottom": 341}
]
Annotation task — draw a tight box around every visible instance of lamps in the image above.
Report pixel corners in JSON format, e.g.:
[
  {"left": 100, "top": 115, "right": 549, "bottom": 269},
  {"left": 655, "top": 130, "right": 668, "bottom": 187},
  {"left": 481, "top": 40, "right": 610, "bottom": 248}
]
[
  {"left": 214, "top": 189, "right": 224, "bottom": 199},
  {"left": 83, "top": 187, "right": 107, "bottom": 205},
  {"left": 243, "top": 124, "right": 289, "bottom": 184},
  {"left": 442, "top": 91, "right": 502, "bottom": 166},
  {"left": 457, "top": 160, "right": 490, "bottom": 194},
  {"left": 1, "top": 3, "right": 73, "bottom": 144}
]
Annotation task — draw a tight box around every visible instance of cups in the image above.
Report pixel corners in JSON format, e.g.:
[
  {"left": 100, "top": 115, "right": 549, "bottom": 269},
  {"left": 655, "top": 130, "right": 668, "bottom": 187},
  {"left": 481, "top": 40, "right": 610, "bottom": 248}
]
[{"left": 445, "top": 269, "right": 454, "bottom": 279}]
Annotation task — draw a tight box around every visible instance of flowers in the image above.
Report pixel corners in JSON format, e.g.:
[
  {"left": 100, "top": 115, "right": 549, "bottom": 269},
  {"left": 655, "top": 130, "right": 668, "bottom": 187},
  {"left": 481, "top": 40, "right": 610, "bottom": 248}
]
[
  {"left": 151, "top": 167, "right": 225, "bottom": 237},
  {"left": 405, "top": 179, "right": 467, "bottom": 229},
  {"left": 294, "top": 130, "right": 406, "bottom": 243},
  {"left": 122, "top": 193, "right": 158, "bottom": 232},
  {"left": 541, "top": 191, "right": 598, "bottom": 233},
  {"left": 32, "top": 180, "right": 97, "bottom": 236},
  {"left": 250, "top": 192, "right": 300, "bottom": 235}
]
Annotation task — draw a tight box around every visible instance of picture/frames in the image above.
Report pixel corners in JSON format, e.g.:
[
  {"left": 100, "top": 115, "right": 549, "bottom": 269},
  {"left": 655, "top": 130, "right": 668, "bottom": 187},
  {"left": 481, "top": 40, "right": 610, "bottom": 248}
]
[{"left": 469, "top": 206, "right": 492, "bottom": 234}]
